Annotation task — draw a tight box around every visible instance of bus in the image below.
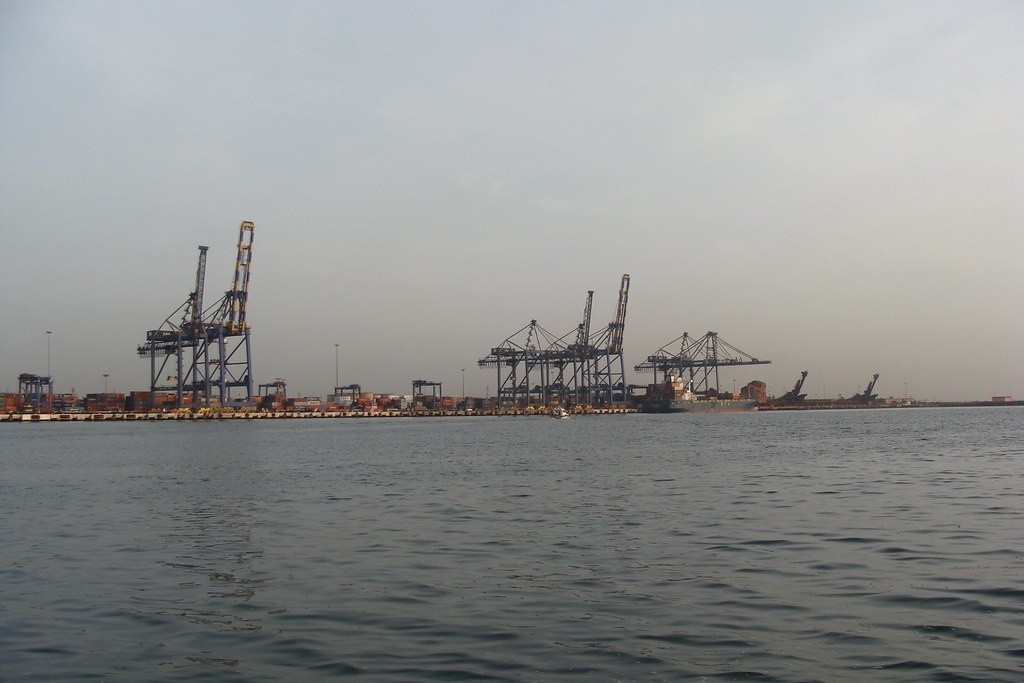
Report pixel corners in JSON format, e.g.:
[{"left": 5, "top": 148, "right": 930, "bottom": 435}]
[
  {"left": 294, "top": 407, "right": 306, "bottom": 411},
  {"left": 388, "top": 409, "right": 402, "bottom": 414}
]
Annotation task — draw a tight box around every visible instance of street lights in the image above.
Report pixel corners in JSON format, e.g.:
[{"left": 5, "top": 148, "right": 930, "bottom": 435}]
[
  {"left": 103, "top": 374, "right": 109, "bottom": 393},
  {"left": 46, "top": 331, "right": 53, "bottom": 407},
  {"left": 334, "top": 344, "right": 340, "bottom": 387},
  {"left": 462, "top": 368, "right": 466, "bottom": 399},
  {"left": 733, "top": 379, "right": 736, "bottom": 393}
]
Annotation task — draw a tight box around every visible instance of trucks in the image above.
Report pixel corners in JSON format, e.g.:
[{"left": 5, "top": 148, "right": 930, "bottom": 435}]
[{"left": 461, "top": 407, "right": 473, "bottom": 411}]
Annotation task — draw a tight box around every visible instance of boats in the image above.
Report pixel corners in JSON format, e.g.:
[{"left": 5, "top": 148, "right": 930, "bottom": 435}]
[{"left": 550, "top": 406, "right": 569, "bottom": 419}]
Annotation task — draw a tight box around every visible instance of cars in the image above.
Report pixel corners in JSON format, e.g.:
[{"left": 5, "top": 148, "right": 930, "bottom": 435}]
[{"left": 352, "top": 405, "right": 370, "bottom": 412}]
